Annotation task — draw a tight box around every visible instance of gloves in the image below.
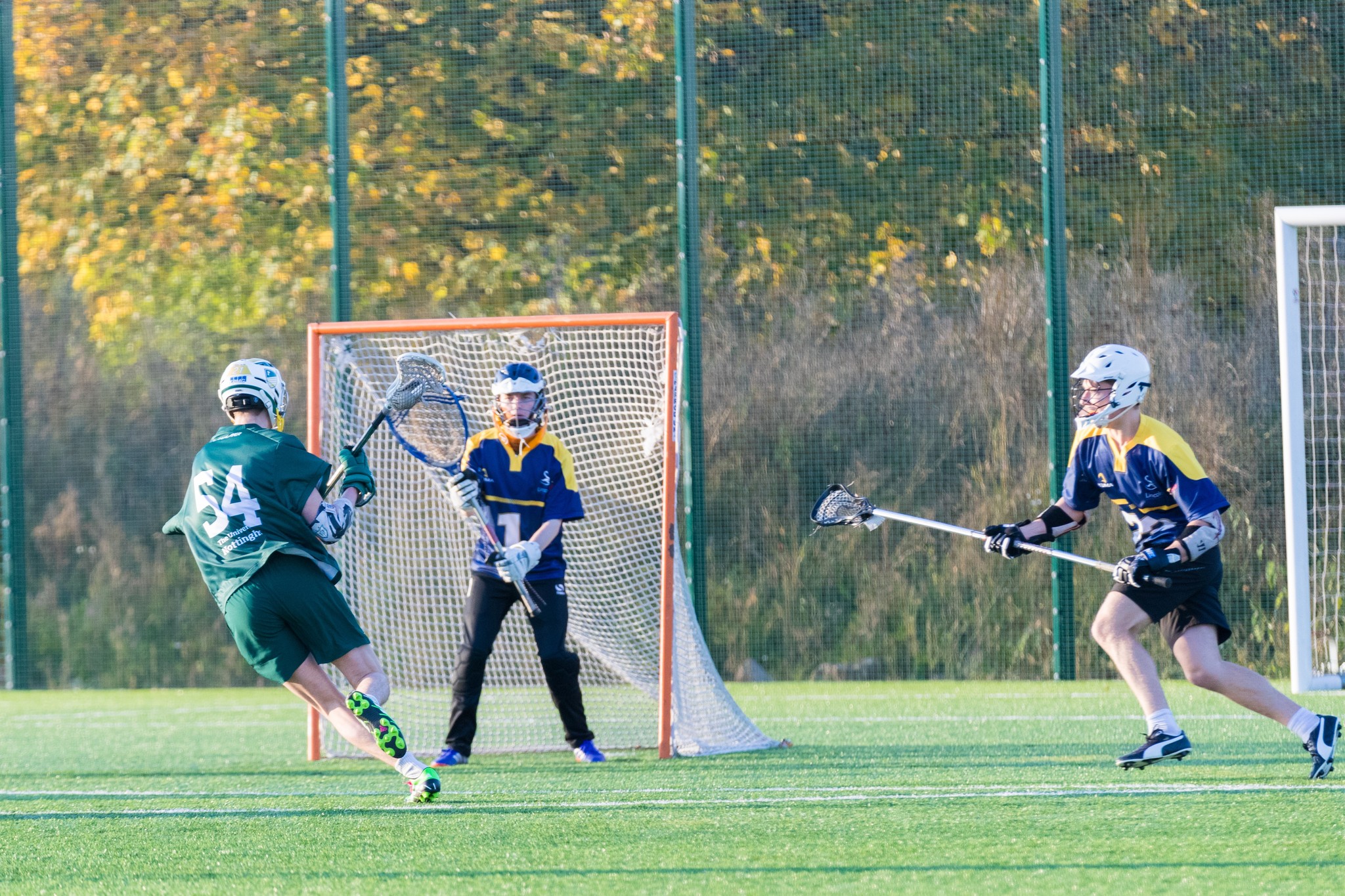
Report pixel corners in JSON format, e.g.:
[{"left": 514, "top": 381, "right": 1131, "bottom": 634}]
[
  {"left": 445, "top": 472, "right": 479, "bottom": 511},
  {"left": 1112, "top": 547, "right": 1181, "bottom": 588},
  {"left": 337, "top": 444, "right": 376, "bottom": 507},
  {"left": 494, "top": 540, "right": 541, "bottom": 582},
  {"left": 982, "top": 519, "right": 1041, "bottom": 560}
]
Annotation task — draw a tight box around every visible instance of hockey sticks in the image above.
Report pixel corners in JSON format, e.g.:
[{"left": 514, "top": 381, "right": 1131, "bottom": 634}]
[
  {"left": 810, "top": 485, "right": 1175, "bottom": 592},
  {"left": 384, "top": 381, "right": 538, "bottom": 619},
  {"left": 295, "top": 354, "right": 448, "bottom": 506}
]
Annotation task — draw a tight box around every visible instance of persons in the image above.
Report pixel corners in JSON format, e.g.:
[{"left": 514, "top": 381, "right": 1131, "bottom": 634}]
[
  {"left": 434, "top": 365, "right": 611, "bottom": 766},
  {"left": 158, "top": 355, "right": 441, "bottom": 807},
  {"left": 983, "top": 344, "right": 1341, "bottom": 782}
]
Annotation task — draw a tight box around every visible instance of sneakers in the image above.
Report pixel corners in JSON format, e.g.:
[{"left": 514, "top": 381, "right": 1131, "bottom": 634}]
[
  {"left": 345, "top": 691, "right": 407, "bottom": 758},
  {"left": 1116, "top": 724, "right": 1192, "bottom": 771},
  {"left": 402, "top": 767, "right": 441, "bottom": 804},
  {"left": 1303, "top": 714, "right": 1342, "bottom": 780},
  {"left": 431, "top": 748, "right": 468, "bottom": 767},
  {"left": 573, "top": 740, "right": 606, "bottom": 764}
]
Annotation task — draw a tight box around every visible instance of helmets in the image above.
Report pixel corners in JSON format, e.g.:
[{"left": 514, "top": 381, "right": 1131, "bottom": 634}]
[
  {"left": 491, "top": 363, "right": 546, "bottom": 439},
  {"left": 218, "top": 358, "right": 284, "bottom": 432},
  {"left": 1069, "top": 343, "right": 1151, "bottom": 430}
]
